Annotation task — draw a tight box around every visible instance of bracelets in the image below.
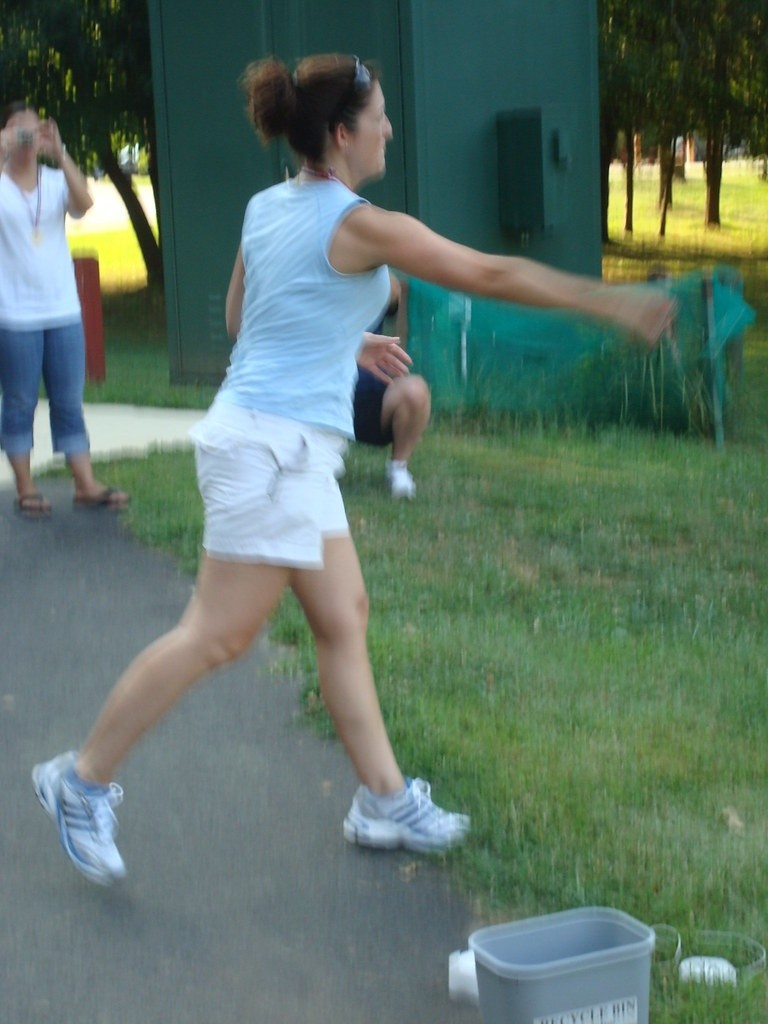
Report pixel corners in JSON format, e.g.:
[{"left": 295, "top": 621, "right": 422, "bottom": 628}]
[{"left": 54, "top": 144, "right": 67, "bottom": 161}]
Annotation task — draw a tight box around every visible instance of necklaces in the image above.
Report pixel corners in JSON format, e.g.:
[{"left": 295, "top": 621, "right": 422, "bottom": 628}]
[{"left": 299, "top": 164, "right": 356, "bottom": 194}]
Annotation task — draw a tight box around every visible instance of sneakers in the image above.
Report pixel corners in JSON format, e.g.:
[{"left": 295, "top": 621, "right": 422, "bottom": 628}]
[
  {"left": 342, "top": 776, "right": 473, "bottom": 854},
  {"left": 31, "top": 748, "right": 123, "bottom": 885}
]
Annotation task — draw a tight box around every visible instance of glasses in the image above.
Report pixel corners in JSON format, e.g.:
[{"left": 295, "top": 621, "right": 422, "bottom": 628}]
[{"left": 329, "top": 53, "right": 371, "bottom": 133}]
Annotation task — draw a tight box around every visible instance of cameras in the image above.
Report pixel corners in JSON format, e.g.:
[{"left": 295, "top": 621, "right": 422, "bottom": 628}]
[{"left": 16, "top": 128, "right": 35, "bottom": 146}]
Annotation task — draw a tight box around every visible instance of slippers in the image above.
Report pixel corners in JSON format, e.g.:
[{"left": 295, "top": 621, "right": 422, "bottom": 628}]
[
  {"left": 72, "top": 483, "right": 131, "bottom": 509},
  {"left": 13, "top": 494, "right": 52, "bottom": 517}
]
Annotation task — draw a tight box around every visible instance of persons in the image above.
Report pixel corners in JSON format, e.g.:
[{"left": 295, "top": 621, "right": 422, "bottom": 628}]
[
  {"left": 333, "top": 270, "right": 431, "bottom": 500},
  {"left": 33, "top": 56, "right": 679, "bottom": 889},
  {"left": 0, "top": 101, "right": 132, "bottom": 518}
]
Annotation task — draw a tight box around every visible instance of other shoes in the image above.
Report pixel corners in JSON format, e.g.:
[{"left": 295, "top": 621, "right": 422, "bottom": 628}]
[{"left": 386, "top": 466, "right": 417, "bottom": 500}]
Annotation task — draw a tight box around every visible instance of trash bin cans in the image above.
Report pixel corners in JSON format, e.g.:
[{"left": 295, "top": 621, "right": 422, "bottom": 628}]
[
  {"left": 72, "top": 256, "right": 106, "bottom": 383},
  {"left": 467, "top": 904, "right": 655, "bottom": 1024}
]
